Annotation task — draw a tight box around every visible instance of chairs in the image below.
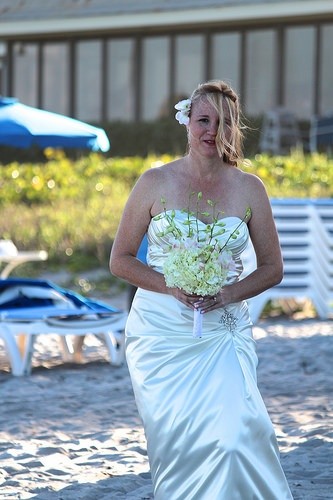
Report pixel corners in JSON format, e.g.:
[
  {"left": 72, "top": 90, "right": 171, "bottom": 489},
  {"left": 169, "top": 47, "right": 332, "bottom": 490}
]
[{"left": 0, "top": 240, "right": 128, "bottom": 377}]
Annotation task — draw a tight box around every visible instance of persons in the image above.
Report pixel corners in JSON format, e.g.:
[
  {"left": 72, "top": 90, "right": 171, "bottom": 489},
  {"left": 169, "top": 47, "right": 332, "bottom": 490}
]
[{"left": 110, "top": 80, "right": 296, "bottom": 500}]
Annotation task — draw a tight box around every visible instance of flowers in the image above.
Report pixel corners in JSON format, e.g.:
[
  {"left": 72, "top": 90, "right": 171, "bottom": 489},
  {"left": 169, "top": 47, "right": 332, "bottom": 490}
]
[
  {"left": 152, "top": 181, "right": 252, "bottom": 340},
  {"left": 174, "top": 98, "right": 192, "bottom": 125}
]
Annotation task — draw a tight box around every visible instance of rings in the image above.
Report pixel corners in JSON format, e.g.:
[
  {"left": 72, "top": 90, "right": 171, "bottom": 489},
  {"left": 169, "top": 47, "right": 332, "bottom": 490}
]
[{"left": 214, "top": 296, "right": 217, "bottom": 302}]
